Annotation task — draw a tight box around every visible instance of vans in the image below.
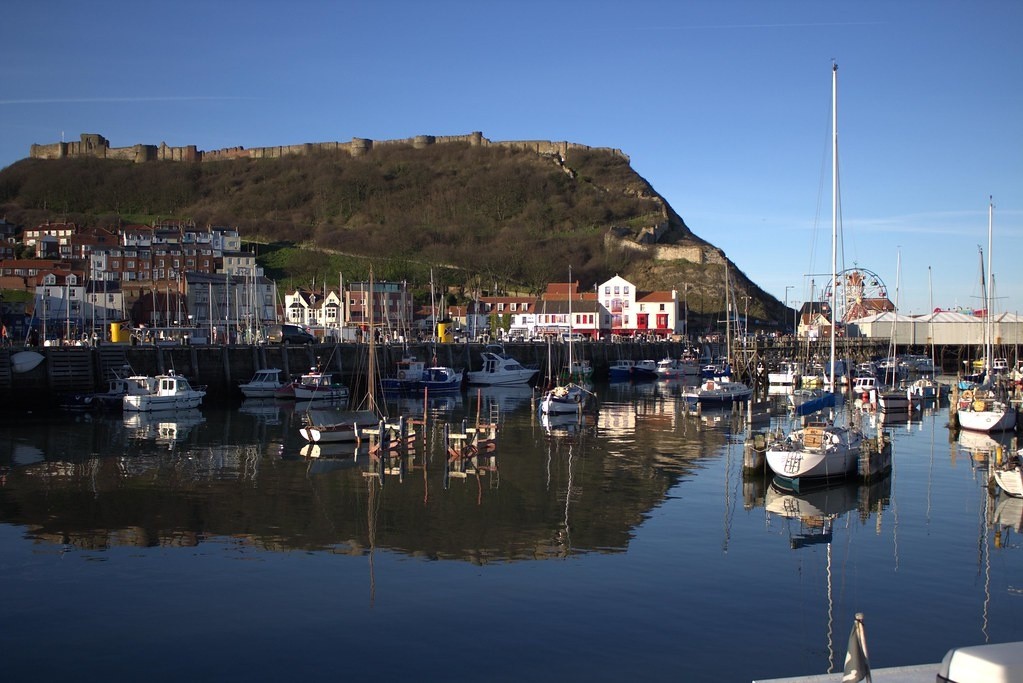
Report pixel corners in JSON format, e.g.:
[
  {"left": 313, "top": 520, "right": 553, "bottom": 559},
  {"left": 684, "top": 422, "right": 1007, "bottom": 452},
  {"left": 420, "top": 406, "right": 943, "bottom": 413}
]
[{"left": 561, "top": 332, "right": 588, "bottom": 342}]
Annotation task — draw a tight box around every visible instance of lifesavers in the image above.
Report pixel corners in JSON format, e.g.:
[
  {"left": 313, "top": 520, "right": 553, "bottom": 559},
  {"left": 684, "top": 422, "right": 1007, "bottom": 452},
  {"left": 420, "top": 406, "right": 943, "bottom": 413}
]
[
  {"left": 398, "top": 371, "right": 406, "bottom": 380},
  {"left": 573, "top": 394, "right": 582, "bottom": 403},
  {"left": 962, "top": 390, "right": 973, "bottom": 399}
]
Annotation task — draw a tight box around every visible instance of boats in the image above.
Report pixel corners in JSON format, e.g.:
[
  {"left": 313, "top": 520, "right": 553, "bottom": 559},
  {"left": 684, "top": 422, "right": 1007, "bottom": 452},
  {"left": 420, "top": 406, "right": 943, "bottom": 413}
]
[
  {"left": 375, "top": 391, "right": 463, "bottom": 422},
  {"left": 469, "top": 383, "right": 535, "bottom": 416},
  {"left": 73, "top": 348, "right": 206, "bottom": 413},
  {"left": 122, "top": 408, "right": 209, "bottom": 452},
  {"left": 293, "top": 398, "right": 347, "bottom": 428},
  {"left": 990, "top": 495, "right": 1023, "bottom": 548},
  {"left": 239, "top": 398, "right": 297, "bottom": 428}
]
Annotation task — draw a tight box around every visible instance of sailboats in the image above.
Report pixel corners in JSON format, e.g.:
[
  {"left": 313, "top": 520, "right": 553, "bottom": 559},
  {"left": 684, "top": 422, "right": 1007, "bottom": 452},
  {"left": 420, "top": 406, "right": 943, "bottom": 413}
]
[
  {"left": 741, "top": 473, "right": 891, "bottom": 672},
  {"left": 297, "top": 442, "right": 389, "bottom": 605},
  {"left": 541, "top": 412, "right": 590, "bottom": 547},
  {"left": 236, "top": 56, "right": 1022, "bottom": 501}
]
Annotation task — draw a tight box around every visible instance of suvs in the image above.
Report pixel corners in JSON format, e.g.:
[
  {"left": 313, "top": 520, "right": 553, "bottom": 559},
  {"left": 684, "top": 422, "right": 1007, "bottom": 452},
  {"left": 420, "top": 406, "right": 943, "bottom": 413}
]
[{"left": 265, "top": 323, "right": 318, "bottom": 346}]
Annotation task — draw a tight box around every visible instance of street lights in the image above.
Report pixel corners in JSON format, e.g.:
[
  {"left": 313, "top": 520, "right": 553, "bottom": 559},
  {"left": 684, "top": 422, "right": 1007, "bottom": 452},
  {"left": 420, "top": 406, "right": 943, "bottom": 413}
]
[
  {"left": 739, "top": 295, "right": 752, "bottom": 337},
  {"left": 784, "top": 286, "right": 795, "bottom": 332},
  {"left": 593, "top": 280, "right": 597, "bottom": 342},
  {"left": 568, "top": 264, "right": 572, "bottom": 341}
]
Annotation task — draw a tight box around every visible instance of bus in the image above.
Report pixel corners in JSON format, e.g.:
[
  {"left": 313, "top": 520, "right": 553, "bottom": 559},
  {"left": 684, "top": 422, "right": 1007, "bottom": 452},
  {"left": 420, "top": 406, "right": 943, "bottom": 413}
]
[{"left": 132, "top": 326, "right": 208, "bottom": 346}]
